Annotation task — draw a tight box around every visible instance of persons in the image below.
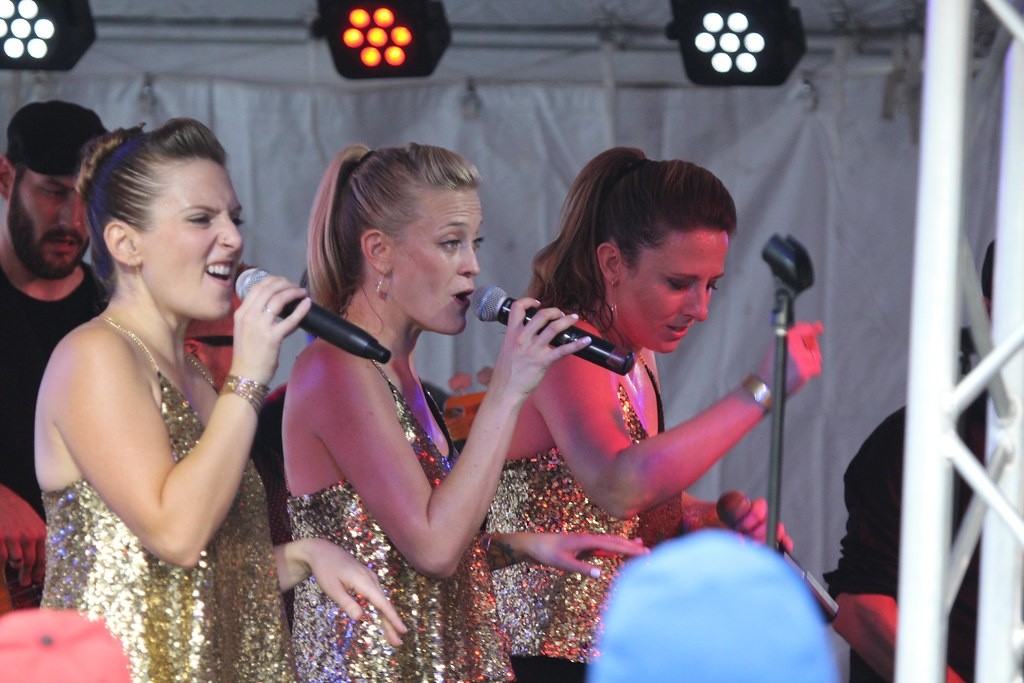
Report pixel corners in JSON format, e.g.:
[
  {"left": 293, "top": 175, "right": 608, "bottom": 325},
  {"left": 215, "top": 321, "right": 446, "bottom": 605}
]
[
  {"left": 280, "top": 142, "right": 650, "bottom": 683},
  {"left": 252, "top": 271, "right": 464, "bottom": 630},
  {"left": 481, "top": 145, "right": 823, "bottom": 683},
  {"left": 588, "top": 532, "right": 836, "bottom": 682},
  {"left": 1, "top": 99, "right": 108, "bottom": 587},
  {"left": 830, "top": 237, "right": 994, "bottom": 683},
  {"left": 34, "top": 117, "right": 406, "bottom": 683},
  {"left": 183, "top": 290, "right": 243, "bottom": 390}
]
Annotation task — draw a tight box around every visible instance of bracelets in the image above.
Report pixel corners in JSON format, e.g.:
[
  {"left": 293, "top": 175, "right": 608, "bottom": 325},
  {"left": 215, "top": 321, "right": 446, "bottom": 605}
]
[
  {"left": 222, "top": 375, "right": 270, "bottom": 416},
  {"left": 744, "top": 374, "right": 774, "bottom": 410}
]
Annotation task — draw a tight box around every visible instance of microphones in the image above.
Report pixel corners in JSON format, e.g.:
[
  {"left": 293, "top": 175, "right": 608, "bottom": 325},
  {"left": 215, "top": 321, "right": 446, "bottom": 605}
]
[
  {"left": 472, "top": 284, "right": 634, "bottom": 376},
  {"left": 235, "top": 268, "right": 391, "bottom": 364}
]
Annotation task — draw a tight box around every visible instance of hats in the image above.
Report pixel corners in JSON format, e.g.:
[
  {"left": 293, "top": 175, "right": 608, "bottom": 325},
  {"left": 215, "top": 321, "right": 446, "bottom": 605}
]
[{"left": 4, "top": 98, "right": 109, "bottom": 178}]
